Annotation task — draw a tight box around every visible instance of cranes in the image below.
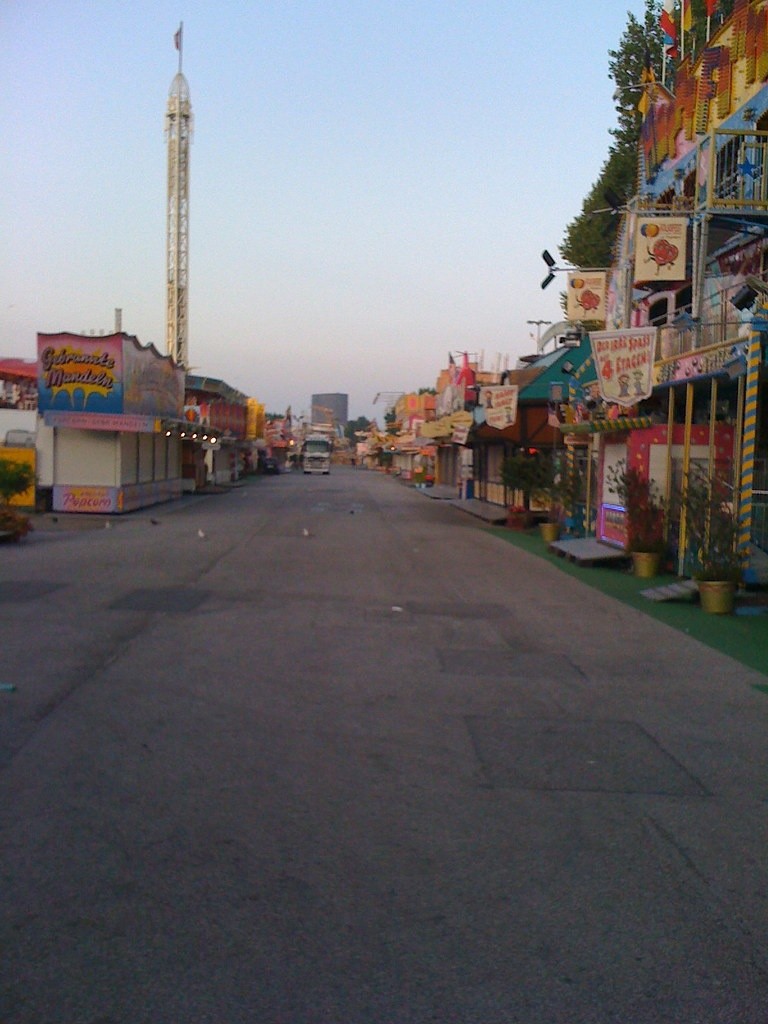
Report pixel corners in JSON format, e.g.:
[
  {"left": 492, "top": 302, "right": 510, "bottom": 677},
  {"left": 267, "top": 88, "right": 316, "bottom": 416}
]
[{"left": 311, "top": 404, "right": 337, "bottom": 425}]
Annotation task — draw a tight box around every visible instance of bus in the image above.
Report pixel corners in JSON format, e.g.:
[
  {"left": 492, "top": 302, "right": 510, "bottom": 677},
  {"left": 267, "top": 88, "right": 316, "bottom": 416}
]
[{"left": 301, "top": 434, "right": 333, "bottom": 475}]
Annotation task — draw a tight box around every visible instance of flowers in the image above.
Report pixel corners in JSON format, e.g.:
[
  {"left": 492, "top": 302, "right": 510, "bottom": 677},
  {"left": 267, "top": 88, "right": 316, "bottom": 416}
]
[
  {"left": 536, "top": 499, "right": 564, "bottom": 523},
  {"left": 606, "top": 461, "right": 666, "bottom": 553}
]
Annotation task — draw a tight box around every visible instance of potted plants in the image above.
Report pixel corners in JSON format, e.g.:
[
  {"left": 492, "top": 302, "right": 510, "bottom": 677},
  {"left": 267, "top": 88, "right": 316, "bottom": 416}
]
[{"left": 683, "top": 475, "right": 745, "bottom": 613}]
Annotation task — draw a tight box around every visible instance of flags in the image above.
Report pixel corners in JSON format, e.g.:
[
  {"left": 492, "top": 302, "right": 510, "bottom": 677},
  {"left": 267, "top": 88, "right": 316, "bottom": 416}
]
[
  {"left": 637, "top": 45, "right": 657, "bottom": 114},
  {"left": 705, "top": 0, "right": 716, "bottom": 14},
  {"left": 683, "top": 0, "right": 697, "bottom": 35},
  {"left": 659, "top": 0, "right": 678, "bottom": 59}
]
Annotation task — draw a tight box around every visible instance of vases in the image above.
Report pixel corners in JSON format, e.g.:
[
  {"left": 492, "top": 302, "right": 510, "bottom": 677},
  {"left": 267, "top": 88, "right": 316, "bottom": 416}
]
[
  {"left": 539, "top": 524, "right": 560, "bottom": 542},
  {"left": 630, "top": 552, "right": 658, "bottom": 577}
]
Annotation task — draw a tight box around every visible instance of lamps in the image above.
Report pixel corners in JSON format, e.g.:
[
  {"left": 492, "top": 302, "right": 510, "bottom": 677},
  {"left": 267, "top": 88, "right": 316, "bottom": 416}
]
[
  {"left": 727, "top": 285, "right": 763, "bottom": 316},
  {"left": 561, "top": 360, "right": 578, "bottom": 378}
]
